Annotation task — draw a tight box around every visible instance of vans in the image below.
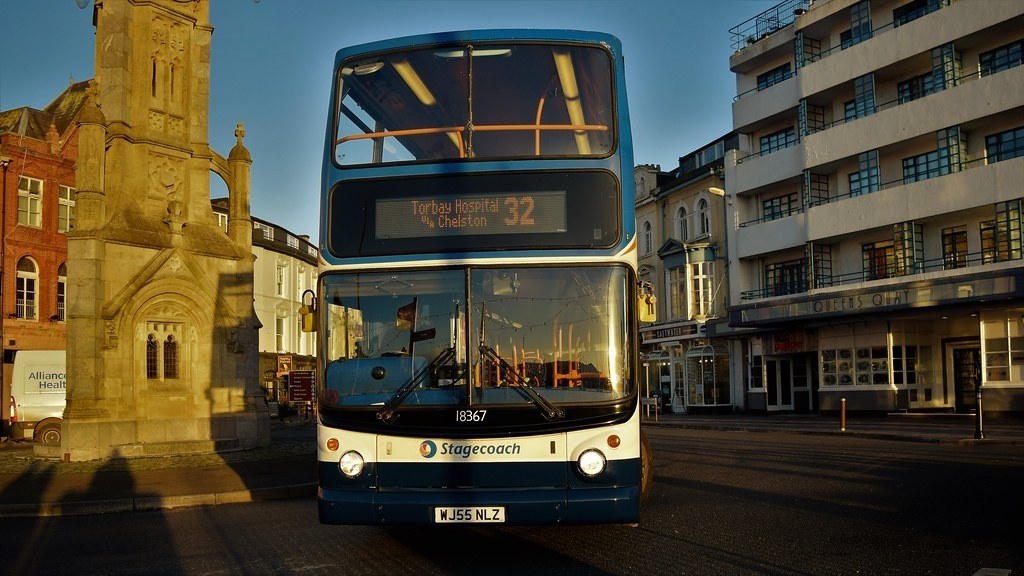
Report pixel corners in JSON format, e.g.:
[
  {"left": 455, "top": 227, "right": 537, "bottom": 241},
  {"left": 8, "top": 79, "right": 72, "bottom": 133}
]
[{"left": 8, "top": 349, "right": 67, "bottom": 448}]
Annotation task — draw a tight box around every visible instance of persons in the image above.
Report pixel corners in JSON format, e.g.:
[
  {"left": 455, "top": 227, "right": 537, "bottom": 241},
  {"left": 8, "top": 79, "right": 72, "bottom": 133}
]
[{"left": 378, "top": 294, "right": 448, "bottom": 367}]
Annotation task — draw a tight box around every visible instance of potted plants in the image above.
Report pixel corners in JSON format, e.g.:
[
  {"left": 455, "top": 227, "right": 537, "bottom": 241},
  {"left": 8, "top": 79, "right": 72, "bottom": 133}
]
[{"left": 747, "top": 36, "right": 755, "bottom": 46}]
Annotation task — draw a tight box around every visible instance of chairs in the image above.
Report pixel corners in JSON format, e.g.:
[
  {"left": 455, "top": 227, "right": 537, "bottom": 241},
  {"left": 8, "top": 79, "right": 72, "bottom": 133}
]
[{"left": 529, "top": 349, "right": 609, "bottom": 389}]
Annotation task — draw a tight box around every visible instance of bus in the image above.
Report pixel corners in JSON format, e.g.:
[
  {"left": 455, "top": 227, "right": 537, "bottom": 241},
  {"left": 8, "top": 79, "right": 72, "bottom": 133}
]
[{"left": 297, "top": 28, "right": 658, "bottom": 526}]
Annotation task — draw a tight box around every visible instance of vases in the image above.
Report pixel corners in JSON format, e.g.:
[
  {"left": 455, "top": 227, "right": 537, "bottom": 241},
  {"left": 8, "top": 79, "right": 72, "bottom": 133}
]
[{"left": 793, "top": 9, "right": 808, "bottom": 15}]
